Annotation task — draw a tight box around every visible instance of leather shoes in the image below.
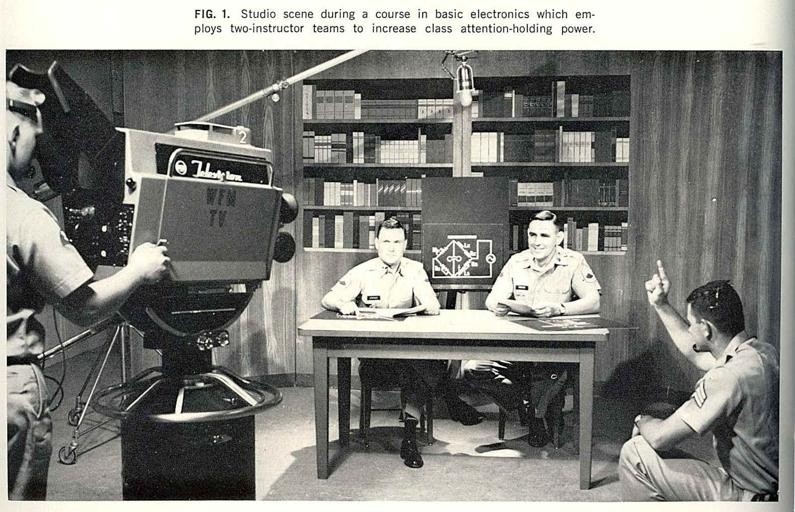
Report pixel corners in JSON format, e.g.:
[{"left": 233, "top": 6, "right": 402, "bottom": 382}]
[
  {"left": 447, "top": 400, "right": 486, "bottom": 426},
  {"left": 518, "top": 403, "right": 531, "bottom": 425},
  {"left": 400, "top": 440, "right": 424, "bottom": 468},
  {"left": 528, "top": 418, "right": 549, "bottom": 447}
]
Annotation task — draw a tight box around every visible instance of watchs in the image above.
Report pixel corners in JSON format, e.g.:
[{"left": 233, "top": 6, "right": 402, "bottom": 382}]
[
  {"left": 633, "top": 414, "right": 643, "bottom": 428},
  {"left": 559, "top": 302, "right": 566, "bottom": 316}
]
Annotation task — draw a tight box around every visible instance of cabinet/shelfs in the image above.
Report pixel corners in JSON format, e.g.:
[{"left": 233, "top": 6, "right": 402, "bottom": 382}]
[
  {"left": 290, "top": 73, "right": 462, "bottom": 386},
  {"left": 464, "top": 72, "right": 640, "bottom": 398}
]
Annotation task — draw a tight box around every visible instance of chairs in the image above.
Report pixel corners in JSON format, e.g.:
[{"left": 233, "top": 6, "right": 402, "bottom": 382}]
[
  {"left": 356, "top": 293, "right": 437, "bottom": 444},
  {"left": 493, "top": 364, "right": 580, "bottom": 446}
]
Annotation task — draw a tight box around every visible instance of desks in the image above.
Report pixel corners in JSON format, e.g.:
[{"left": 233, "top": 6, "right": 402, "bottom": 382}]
[{"left": 297, "top": 304, "right": 611, "bottom": 490}]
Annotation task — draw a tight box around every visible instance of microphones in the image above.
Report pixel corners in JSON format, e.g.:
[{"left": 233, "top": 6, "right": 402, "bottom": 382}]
[{"left": 458, "top": 64, "right": 472, "bottom": 107}]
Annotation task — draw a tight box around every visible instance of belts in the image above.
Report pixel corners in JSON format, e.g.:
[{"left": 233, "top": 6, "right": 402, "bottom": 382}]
[{"left": 7, "top": 355, "right": 32, "bottom": 365}]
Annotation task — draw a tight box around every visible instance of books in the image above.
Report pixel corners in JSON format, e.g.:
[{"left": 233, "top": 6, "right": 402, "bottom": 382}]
[
  {"left": 470, "top": 79, "right": 630, "bottom": 118},
  {"left": 302, "top": 84, "right": 453, "bottom": 119},
  {"left": 511, "top": 177, "right": 629, "bottom": 207},
  {"left": 471, "top": 122, "right": 629, "bottom": 163},
  {"left": 563, "top": 215, "right": 627, "bottom": 252},
  {"left": 303, "top": 210, "right": 421, "bottom": 250},
  {"left": 304, "top": 177, "right": 422, "bottom": 207},
  {"left": 303, "top": 127, "right": 454, "bottom": 164},
  {"left": 510, "top": 223, "right": 529, "bottom": 250}
]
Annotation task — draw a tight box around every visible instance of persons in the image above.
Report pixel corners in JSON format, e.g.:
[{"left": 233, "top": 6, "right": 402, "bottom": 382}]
[
  {"left": 618, "top": 258, "right": 779, "bottom": 502},
  {"left": 6, "top": 81, "right": 170, "bottom": 501},
  {"left": 319, "top": 217, "right": 487, "bottom": 468},
  {"left": 465, "top": 209, "right": 602, "bottom": 447}
]
